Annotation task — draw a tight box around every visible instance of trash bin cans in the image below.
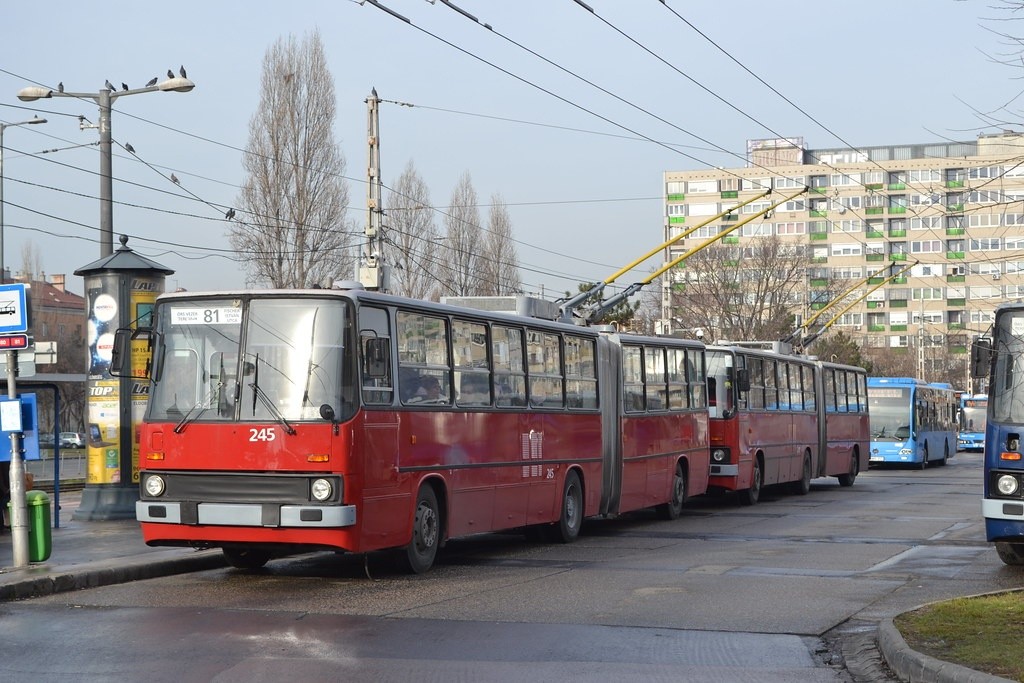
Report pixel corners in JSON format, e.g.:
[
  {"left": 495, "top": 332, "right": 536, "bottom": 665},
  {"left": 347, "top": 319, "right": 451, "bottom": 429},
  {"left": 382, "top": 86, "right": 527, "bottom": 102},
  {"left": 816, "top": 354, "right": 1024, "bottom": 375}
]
[{"left": 7, "top": 490, "right": 51, "bottom": 561}]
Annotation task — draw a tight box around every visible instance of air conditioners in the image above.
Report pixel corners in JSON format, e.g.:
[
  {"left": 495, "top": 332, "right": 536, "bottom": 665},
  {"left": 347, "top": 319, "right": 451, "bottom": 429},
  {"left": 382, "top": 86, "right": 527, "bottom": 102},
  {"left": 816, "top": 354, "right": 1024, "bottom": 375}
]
[
  {"left": 853, "top": 325, "right": 861, "bottom": 331},
  {"left": 876, "top": 302, "right": 884, "bottom": 308},
  {"left": 991, "top": 274, "right": 1000, "bottom": 280}
]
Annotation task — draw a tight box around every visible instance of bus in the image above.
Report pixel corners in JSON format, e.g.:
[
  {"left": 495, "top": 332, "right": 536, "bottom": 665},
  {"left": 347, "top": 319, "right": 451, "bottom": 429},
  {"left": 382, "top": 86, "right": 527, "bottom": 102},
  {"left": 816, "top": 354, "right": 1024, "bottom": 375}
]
[
  {"left": 971, "top": 300, "right": 1024, "bottom": 565},
  {"left": 698, "top": 261, "right": 918, "bottom": 507},
  {"left": 108, "top": 183, "right": 812, "bottom": 574},
  {"left": 956, "top": 394, "right": 988, "bottom": 452},
  {"left": 863, "top": 377, "right": 957, "bottom": 469}
]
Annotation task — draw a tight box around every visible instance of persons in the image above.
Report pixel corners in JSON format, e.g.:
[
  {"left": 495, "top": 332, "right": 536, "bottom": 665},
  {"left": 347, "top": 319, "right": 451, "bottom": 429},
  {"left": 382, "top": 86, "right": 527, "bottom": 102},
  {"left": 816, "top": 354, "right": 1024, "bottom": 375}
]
[
  {"left": 461, "top": 380, "right": 482, "bottom": 406},
  {"left": 627, "top": 391, "right": 643, "bottom": 411},
  {"left": 568, "top": 391, "right": 580, "bottom": 407},
  {"left": 419, "top": 374, "right": 449, "bottom": 405},
  {"left": 499, "top": 386, "right": 523, "bottom": 406}
]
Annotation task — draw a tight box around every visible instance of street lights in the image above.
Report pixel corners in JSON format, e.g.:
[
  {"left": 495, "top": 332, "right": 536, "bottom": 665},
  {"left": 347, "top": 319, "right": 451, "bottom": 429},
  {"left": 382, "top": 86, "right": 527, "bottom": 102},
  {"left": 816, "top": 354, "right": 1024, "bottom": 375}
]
[
  {"left": 15, "top": 76, "right": 197, "bottom": 255},
  {"left": 0, "top": 119, "right": 48, "bottom": 290}
]
